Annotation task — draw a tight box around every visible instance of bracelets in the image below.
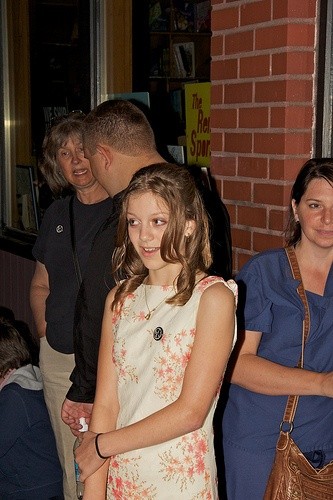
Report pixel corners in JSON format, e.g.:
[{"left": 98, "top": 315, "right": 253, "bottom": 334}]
[{"left": 94, "top": 433, "right": 109, "bottom": 459}]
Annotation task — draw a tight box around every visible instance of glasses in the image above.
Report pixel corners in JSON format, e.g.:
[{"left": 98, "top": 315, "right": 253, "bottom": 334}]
[{"left": 50, "top": 110, "right": 86, "bottom": 128}]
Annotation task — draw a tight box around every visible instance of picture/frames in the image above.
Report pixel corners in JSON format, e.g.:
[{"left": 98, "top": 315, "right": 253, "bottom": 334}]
[
  {"left": 15, "top": 164, "right": 40, "bottom": 235},
  {"left": 173, "top": 41, "right": 196, "bottom": 78}
]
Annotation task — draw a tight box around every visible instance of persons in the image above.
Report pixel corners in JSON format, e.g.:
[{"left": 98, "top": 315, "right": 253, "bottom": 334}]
[
  {"left": 30, "top": 112, "right": 111, "bottom": 500},
  {"left": 74, "top": 162, "right": 237, "bottom": 500},
  {"left": 223, "top": 156, "right": 333, "bottom": 500},
  {"left": 61, "top": 99, "right": 230, "bottom": 500},
  {"left": 0, "top": 305, "right": 65, "bottom": 500}
]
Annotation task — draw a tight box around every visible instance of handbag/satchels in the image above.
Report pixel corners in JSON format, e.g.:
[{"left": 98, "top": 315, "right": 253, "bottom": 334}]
[{"left": 261, "top": 431, "right": 333, "bottom": 500}]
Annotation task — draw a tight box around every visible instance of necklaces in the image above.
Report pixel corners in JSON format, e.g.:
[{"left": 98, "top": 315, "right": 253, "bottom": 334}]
[{"left": 142, "top": 278, "right": 178, "bottom": 321}]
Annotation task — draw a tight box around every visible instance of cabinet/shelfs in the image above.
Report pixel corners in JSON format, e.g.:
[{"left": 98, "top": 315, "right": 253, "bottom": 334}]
[{"left": 147, "top": 31, "right": 212, "bottom": 93}]
